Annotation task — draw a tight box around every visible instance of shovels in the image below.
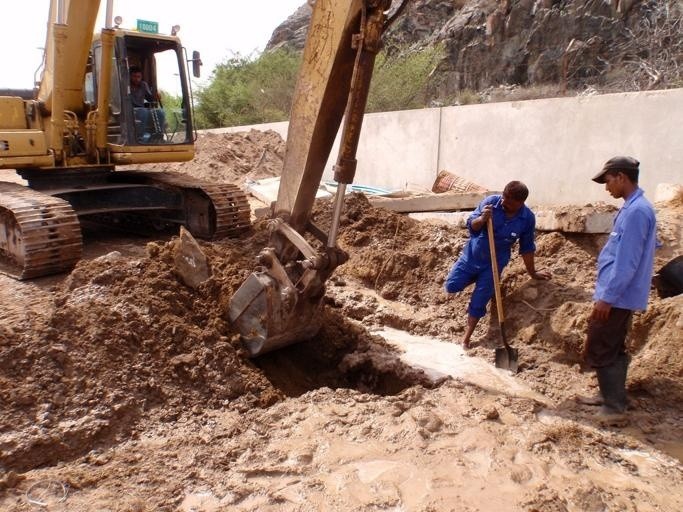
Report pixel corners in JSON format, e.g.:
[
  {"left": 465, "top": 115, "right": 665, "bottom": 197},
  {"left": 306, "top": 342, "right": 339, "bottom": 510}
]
[{"left": 485, "top": 218, "right": 518, "bottom": 375}]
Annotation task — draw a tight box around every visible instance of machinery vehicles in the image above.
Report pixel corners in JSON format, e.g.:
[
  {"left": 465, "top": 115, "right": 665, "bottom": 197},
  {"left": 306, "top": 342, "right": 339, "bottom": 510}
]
[{"left": 0, "top": 0, "right": 413, "bottom": 360}]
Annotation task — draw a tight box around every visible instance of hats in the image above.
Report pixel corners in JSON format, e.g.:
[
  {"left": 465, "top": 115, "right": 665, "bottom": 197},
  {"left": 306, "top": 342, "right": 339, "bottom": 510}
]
[{"left": 592, "top": 156, "right": 640, "bottom": 184}]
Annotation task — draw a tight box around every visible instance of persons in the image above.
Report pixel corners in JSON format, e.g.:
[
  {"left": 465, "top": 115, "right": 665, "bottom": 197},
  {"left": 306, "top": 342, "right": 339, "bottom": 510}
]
[
  {"left": 443, "top": 181, "right": 553, "bottom": 354},
  {"left": 124, "top": 65, "right": 174, "bottom": 145},
  {"left": 583, "top": 155, "right": 658, "bottom": 427}
]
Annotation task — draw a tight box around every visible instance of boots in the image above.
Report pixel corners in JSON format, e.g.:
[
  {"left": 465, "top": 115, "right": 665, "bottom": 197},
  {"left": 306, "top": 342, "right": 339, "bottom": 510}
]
[{"left": 595, "top": 356, "right": 628, "bottom": 428}]
